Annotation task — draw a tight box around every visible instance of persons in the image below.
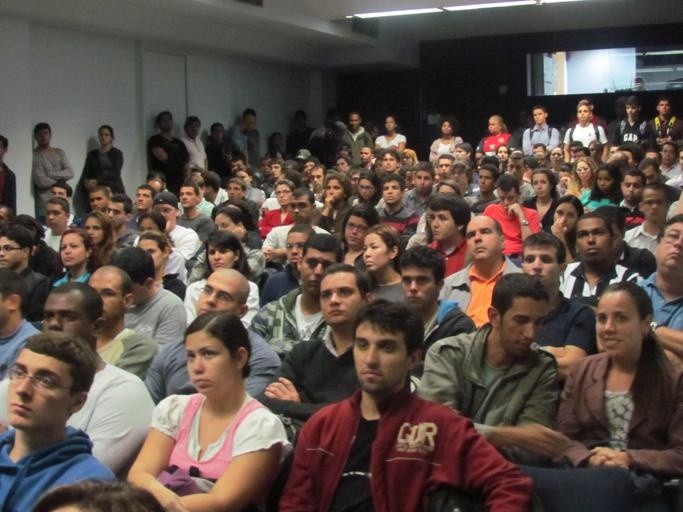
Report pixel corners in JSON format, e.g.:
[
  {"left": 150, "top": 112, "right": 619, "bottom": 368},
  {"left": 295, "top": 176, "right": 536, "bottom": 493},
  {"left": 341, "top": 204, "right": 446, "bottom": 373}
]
[
  {"left": 257, "top": 260, "right": 377, "bottom": 419},
  {"left": 553, "top": 283, "right": 683, "bottom": 510},
  {"left": 0, "top": 278, "right": 156, "bottom": 478},
  {"left": 141, "top": 266, "right": 282, "bottom": 408},
  {"left": 30, "top": 476, "right": 163, "bottom": 512},
  {"left": 414, "top": 273, "right": 568, "bottom": 464},
  {"left": 279, "top": 300, "right": 533, "bottom": 511},
  {"left": 126, "top": 313, "right": 293, "bottom": 512},
  {"left": 0, "top": 330, "right": 117, "bottom": 512},
  {"left": 0, "top": 86, "right": 682, "bottom": 381}
]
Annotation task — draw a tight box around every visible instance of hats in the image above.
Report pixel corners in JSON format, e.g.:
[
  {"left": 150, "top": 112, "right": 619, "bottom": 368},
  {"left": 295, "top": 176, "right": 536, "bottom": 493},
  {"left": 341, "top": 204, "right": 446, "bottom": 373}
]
[
  {"left": 295, "top": 148, "right": 311, "bottom": 161},
  {"left": 153, "top": 191, "right": 179, "bottom": 209}
]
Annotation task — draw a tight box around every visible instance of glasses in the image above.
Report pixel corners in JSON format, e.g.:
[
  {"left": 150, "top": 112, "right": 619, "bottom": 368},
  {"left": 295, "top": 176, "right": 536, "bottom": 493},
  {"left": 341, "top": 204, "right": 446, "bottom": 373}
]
[
  {"left": 0, "top": 246, "right": 21, "bottom": 252},
  {"left": 7, "top": 365, "right": 72, "bottom": 391}
]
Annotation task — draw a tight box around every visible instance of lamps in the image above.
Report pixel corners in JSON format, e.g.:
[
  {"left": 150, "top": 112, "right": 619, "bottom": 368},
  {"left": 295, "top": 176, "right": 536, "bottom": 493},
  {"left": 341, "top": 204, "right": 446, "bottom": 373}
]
[
  {"left": 443, "top": 0, "right": 538, "bottom": 12},
  {"left": 345, "top": 6, "right": 447, "bottom": 19}
]
